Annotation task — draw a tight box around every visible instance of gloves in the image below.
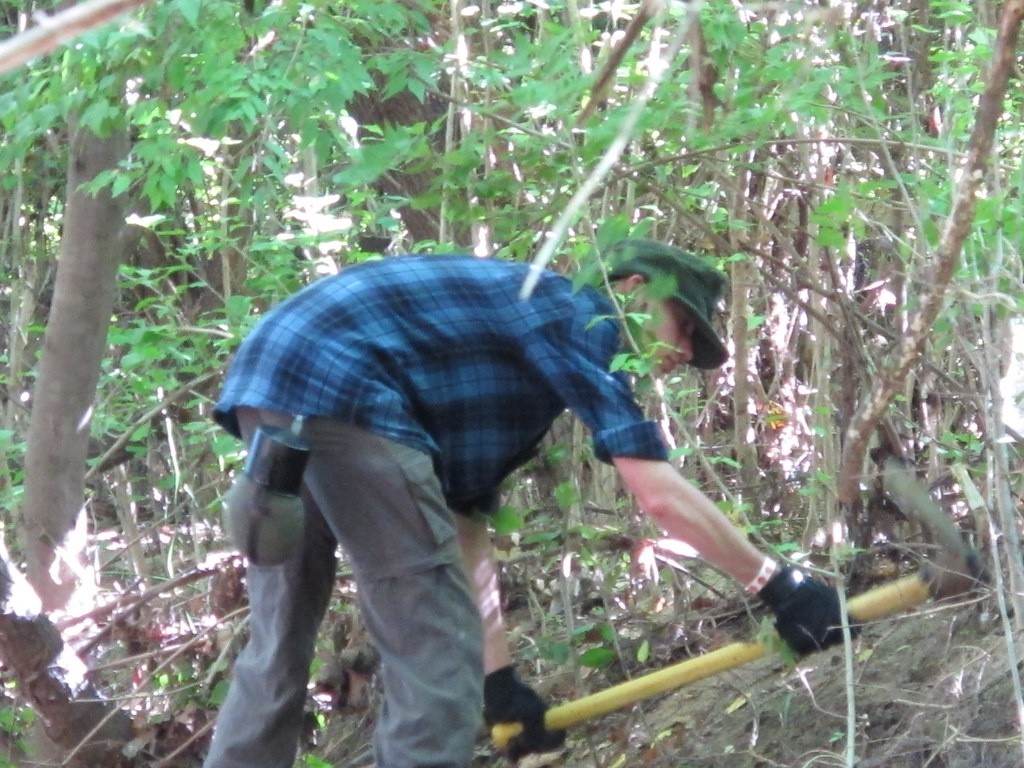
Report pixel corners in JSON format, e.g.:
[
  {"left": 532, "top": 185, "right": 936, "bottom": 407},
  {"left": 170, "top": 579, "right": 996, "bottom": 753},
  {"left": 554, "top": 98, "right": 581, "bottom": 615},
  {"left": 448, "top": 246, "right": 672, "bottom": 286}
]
[
  {"left": 757, "top": 569, "right": 863, "bottom": 658},
  {"left": 480, "top": 668, "right": 568, "bottom": 768}
]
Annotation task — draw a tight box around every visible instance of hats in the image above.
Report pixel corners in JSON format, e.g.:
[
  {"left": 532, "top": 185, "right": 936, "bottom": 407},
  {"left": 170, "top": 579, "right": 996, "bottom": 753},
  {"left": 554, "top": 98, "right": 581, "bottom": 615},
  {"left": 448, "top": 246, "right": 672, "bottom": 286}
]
[{"left": 600, "top": 239, "right": 730, "bottom": 371}]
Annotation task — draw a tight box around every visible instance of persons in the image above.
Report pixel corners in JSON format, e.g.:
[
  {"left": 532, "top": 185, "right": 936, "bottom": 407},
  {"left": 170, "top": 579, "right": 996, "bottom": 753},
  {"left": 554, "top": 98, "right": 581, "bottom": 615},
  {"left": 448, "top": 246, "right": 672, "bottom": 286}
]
[{"left": 202, "top": 237, "right": 860, "bottom": 768}]
